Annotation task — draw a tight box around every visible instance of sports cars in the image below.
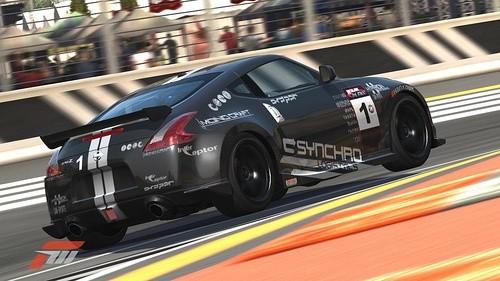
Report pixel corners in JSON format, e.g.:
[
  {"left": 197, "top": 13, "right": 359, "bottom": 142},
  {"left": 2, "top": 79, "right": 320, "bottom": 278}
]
[{"left": 41, "top": 55, "right": 446, "bottom": 252}]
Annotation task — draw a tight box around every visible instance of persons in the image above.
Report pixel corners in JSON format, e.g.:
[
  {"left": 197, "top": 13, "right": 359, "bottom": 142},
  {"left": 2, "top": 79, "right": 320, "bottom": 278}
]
[{"left": 0, "top": 1, "right": 398, "bottom": 89}]
